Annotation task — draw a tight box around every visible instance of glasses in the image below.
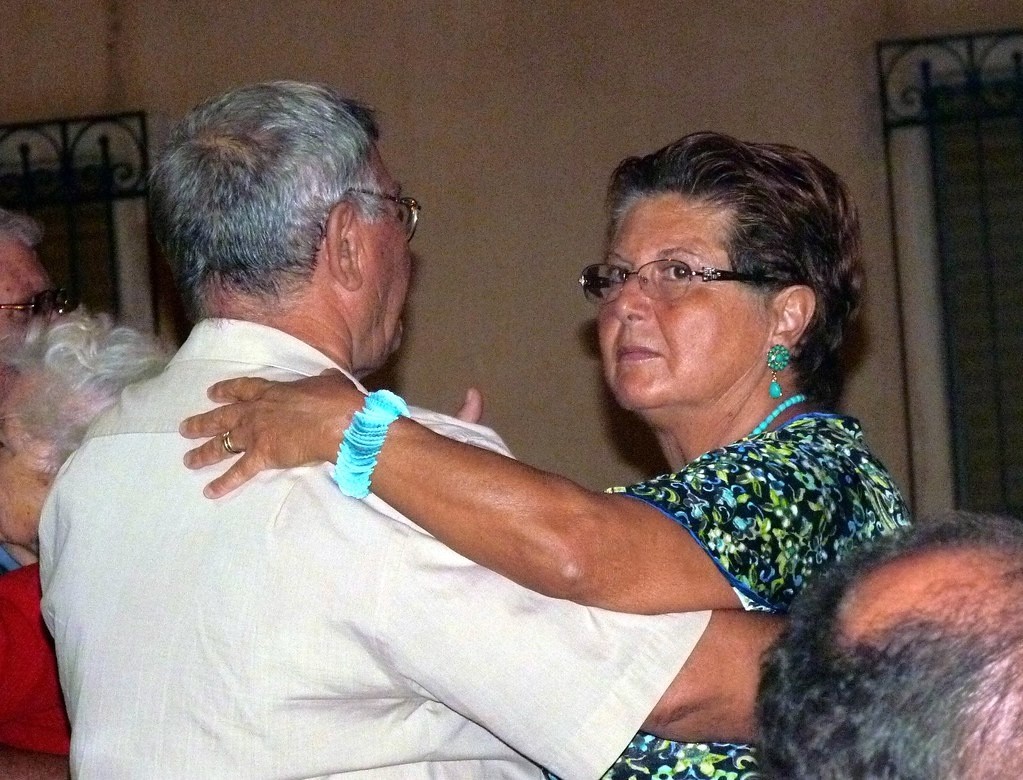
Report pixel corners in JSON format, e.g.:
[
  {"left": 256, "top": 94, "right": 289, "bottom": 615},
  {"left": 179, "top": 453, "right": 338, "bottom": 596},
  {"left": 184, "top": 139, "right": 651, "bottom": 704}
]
[
  {"left": 0, "top": 288, "right": 74, "bottom": 326},
  {"left": 348, "top": 186, "right": 424, "bottom": 242},
  {"left": 578, "top": 257, "right": 787, "bottom": 306}
]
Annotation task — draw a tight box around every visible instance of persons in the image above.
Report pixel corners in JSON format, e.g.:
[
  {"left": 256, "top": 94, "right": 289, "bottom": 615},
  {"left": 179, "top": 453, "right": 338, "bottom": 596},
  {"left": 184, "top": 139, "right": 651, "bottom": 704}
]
[
  {"left": 38, "top": 78, "right": 786, "bottom": 779},
  {"left": 755, "top": 511, "right": 1022, "bottom": 780},
  {"left": 182, "top": 131, "right": 918, "bottom": 779},
  {"left": 1, "top": 307, "right": 181, "bottom": 780},
  {"left": 1, "top": 212, "right": 72, "bottom": 571}
]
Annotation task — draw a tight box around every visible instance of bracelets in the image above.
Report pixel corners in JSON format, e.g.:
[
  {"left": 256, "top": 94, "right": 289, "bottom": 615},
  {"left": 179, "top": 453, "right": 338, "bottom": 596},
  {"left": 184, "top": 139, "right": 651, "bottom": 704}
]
[{"left": 335, "top": 390, "right": 408, "bottom": 500}]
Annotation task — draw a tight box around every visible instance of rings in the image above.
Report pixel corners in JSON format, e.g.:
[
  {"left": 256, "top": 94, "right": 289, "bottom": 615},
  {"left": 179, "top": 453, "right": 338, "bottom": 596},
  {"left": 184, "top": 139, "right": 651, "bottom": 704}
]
[{"left": 220, "top": 431, "right": 242, "bottom": 456}]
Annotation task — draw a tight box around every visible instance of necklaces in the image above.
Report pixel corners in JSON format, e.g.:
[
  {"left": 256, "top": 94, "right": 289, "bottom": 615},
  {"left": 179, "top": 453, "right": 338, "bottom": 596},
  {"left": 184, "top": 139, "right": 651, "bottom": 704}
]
[{"left": 745, "top": 394, "right": 809, "bottom": 439}]
